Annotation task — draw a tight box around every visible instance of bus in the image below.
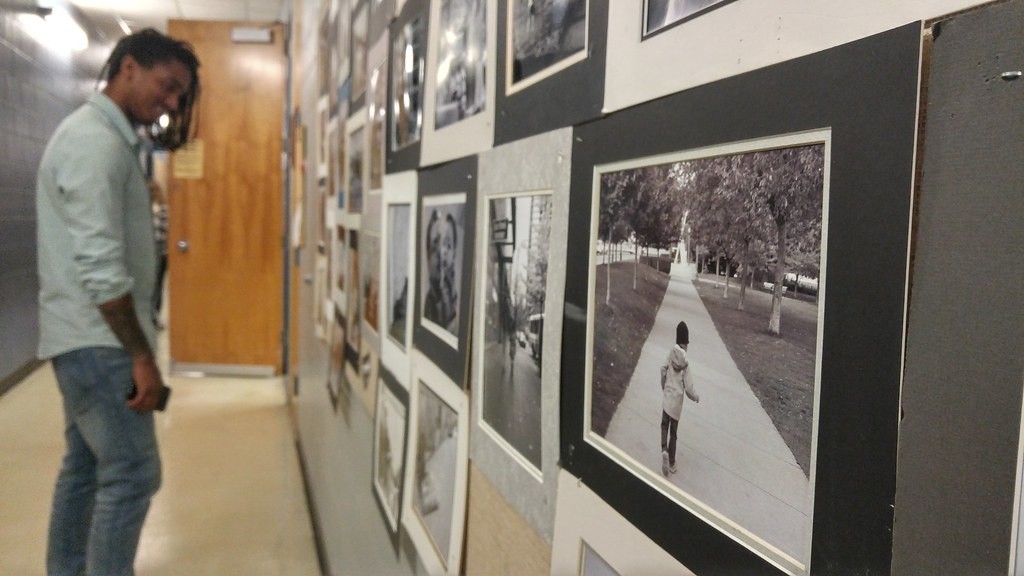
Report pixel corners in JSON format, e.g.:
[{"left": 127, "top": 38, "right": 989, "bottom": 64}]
[{"left": 524, "top": 313, "right": 544, "bottom": 362}]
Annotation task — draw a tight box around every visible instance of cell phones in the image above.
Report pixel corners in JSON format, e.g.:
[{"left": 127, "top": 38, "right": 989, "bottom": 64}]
[{"left": 127, "top": 379, "right": 170, "bottom": 412}]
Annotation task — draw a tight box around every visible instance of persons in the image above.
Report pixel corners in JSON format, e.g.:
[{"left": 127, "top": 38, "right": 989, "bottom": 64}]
[
  {"left": 661, "top": 322, "right": 700, "bottom": 477},
  {"left": 424, "top": 210, "right": 458, "bottom": 339},
  {"left": 35, "top": 29, "right": 203, "bottom": 576}
]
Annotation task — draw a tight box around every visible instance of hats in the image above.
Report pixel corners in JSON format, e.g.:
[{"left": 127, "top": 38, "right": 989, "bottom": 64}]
[{"left": 676, "top": 321, "right": 690, "bottom": 344}]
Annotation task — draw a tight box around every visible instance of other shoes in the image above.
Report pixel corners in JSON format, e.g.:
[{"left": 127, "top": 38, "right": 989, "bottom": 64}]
[
  {"left": 670, "top": 463, "right": 676, "bottom": 473},
  {"left": 662, "top": 452, "right": 670, "bottom": 475}
]
